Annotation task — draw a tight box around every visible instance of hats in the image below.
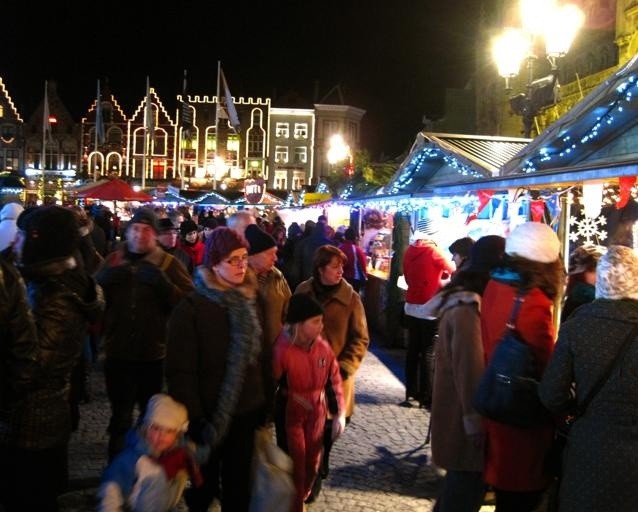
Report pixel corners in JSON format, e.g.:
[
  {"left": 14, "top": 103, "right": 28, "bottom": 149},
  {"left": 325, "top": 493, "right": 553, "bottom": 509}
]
[
  {"left": 202, "top": 228, "right": 249, "bottom": 268},
  {"left": 595, "top": 247, "right": 637, "bottom": 301},
  {"left": 286, "top": 295, "right": 323, "bottom": 322},
  {"left": 568, "top": 244, "right": 607, "bottom": 276},
  {"left": 244, "top": 224, "right": 277, "bottom": 255},
  {"left": 505, "top": 222, "right": 560, "bottom": 263},
  {"left": 17, "top": 206, "right": 81, "bottom": 255},
  {"left": 158, "top": 218, "right": 180, "bottom": 233},
  {"left": 127, "top": 208, "right": 160, "bottom": 234},
  {"left": 143, "top": 395, "right": 187, "bottom": 433},
  {"left": 180, "top": 220, "right": 198, "bottom": 238},
  {"left": 416, "top": 217, "right": 437, "bottom": 234}
]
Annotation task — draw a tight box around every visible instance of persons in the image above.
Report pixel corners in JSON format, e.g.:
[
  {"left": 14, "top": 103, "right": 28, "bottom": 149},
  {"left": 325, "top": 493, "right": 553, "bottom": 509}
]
[
  {"left": 154, "top": 206, "right": 259, "bottom": 267},
  {"left": 450, "top": 237, "right": 475, "bottom": 269},
  {"left": 92, "top": 208, "right": 197, "bottom": 462},
  {"left": 538, "top": 245, "right": 638, "bottom": 512},
  {"left": 294, "top": 245, "right": 369, "bottom": 497},
  {"left": 430, "top": 234, "right": 505, "bottom": 512},
  {"left": 402, "top": 218, "right": 455, "bottom": 410},
  {"left": 256, "top": 212, "right": 285, "bottom": 270},
  {"left": 481, "top": 222, "right": 567, "bottom": 511},
  {"left": 99, "top": 393, "right": 223, "bottom": 512},
  {"left": 280, "top": 215, "right": 369, "bottom": 293},
  {"left": 67, "top": 204, "right": 121, "bottom": 272},
  {"left": 0, "top": 204, "right": 111, "bottom": 512},
  {"left": 274, "top": 294, "right": 345, "bottom": 512},
  {"left": 244, "top": 225, "right": 292, "bottom": 340},
  {"left": 1, "top": 203, "right": 25, "bottom": 265},
  {"left": 170, "top": 227, "right": 275, "bottom": 512},
  {"left": 561, "top": 244, "right": 608, "bottom": 323}
]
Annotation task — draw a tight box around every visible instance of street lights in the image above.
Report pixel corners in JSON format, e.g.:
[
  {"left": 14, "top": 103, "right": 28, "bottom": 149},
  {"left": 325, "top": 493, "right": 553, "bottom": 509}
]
[{"left": 488, "top": 0, "right": 587, "bottom": 137}]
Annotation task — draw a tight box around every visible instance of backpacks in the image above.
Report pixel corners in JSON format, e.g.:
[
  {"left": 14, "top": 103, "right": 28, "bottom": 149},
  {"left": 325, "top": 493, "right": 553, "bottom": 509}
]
[{"left": 96, "top": 261, "right": 172, "bottom": 340}]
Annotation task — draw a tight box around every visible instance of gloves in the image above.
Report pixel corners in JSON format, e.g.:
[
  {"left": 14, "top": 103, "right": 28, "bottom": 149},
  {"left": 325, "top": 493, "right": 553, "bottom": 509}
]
[
  {"left": 96, "top": 263, "right": 133, "bottom": 288},
  {"left": 137, "top": 266, "right": 173, "bottom": 296}
]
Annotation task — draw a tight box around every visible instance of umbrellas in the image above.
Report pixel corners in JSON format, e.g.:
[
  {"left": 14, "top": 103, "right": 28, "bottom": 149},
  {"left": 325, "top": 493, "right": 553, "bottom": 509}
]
[{"left": 65, "top": 171, "right": 158, "bottom": 237}]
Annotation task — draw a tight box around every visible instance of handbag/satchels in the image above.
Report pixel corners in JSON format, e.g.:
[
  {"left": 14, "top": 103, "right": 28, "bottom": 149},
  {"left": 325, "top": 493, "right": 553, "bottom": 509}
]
[{"left": 474, "top": 292, "right": 554, "bottom": 427}]
